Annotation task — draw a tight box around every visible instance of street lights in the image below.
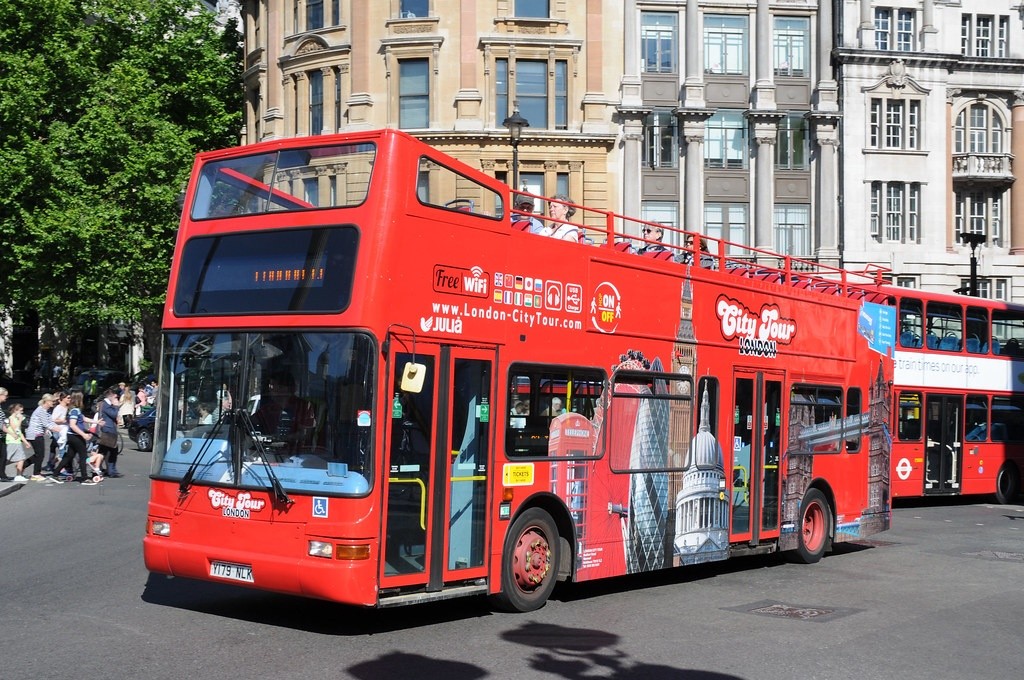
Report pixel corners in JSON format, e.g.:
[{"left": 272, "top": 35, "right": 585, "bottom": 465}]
[
  {"left": 502, "top": 110, "right": 529, "bottom": 221},
  {"left": 954, "top": 231, "right": 992, "bottom": 296}
]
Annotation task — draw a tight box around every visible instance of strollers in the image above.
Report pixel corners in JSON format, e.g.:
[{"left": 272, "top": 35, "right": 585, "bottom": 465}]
[{"left": 53, "top": 427, "right": 104, "bottom": 483}]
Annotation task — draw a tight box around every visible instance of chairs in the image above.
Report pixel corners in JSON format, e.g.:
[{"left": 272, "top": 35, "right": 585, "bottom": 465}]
[
  {"left": 304, "top": 395, "right": 327, "bottom": 452},
  {"left": 900, "top": 329, "right": 1021, "bottom": 356},
  {"left": 447, "top": 200, "right": 867, "bottom": 300}
]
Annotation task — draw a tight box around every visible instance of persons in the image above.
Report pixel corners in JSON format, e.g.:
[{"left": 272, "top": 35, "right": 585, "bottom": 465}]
[
  {"left": 198, "top": 403, "right": 212, "bottom": 424},
  {"left": 212, "top": 390, "right": 232, "bottom": 424},
  {"left": 221, "top": 371, "right": 324, "bottom": 455},
  {"left": 495, "top": 395, "right": 561, "bottom": 428},
  {"left": 633, "top": 223, "right": 669, "bottom": 256},
  {"left": 120, "top": 381, "right": 158, "bottom": 428},
  {"left": 674, "top": 235, "right": 714, "bottom": 270},
  {"left": 539, "top": 195, "right": 578, "bottom": 243},
  {"left": 0, "top": 387, "right": 124, "bottom": 485},
  {"left": 511, "top": 191, "right": 543, "bottom": 233}
]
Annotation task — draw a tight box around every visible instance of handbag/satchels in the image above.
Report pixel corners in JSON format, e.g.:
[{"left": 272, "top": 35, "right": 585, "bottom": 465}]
[{"left": 97, "top": 432, "right": 118, "bottom": 448}]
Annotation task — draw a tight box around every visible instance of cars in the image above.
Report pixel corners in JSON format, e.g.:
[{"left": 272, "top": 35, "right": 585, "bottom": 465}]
[
  {"left": 68, "top": 368, "right": 125, "bottom": 411},
  {"left": 128, "top": 404, "right": 218, "bottom": 452}
]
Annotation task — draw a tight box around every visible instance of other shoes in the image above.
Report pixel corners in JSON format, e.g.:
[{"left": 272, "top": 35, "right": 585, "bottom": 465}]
[
  {"left": 81, "top": 479, "right": 97, "bottom": 485},
  {"left": 49, "top": 475, "right": 64, "bottom": 484},
  {"left": 15, "top": 476, "right": 28, "bottom": 481},
  {"left": 1, "top": 478, "right": 11, "bottom": 482},
  {"left": 7, "top": 477, "right": 14, "bottom": 480},
  {"left": 31, "top": 475, "right": 46, "bottom": 480}
]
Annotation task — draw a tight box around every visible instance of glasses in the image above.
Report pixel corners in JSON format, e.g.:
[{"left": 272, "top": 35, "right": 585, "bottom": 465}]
[
  {"left": 642, "top": 229, "right": 651, "bottom": 233},
  {"left": 685, "top": 241, "right": 693, "bottom": 245},
  {"left": 268, "top": 384, "right": 274, "bottom": 389},
  {"left": 66, "top": 396, "right": 71, "bottom": 399},
  {"left": 519, "top": 206, "right": 532, "bottom": 210}
]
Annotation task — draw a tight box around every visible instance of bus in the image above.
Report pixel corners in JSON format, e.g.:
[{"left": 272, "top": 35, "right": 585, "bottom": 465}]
[{"left": 144, "top": 128, "right": 1024, "bottom": 613}]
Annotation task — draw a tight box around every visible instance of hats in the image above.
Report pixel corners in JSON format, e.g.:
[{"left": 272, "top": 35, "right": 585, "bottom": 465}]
[{"left": 516, "top": 194, "right": 535, "bottom": 206}]
